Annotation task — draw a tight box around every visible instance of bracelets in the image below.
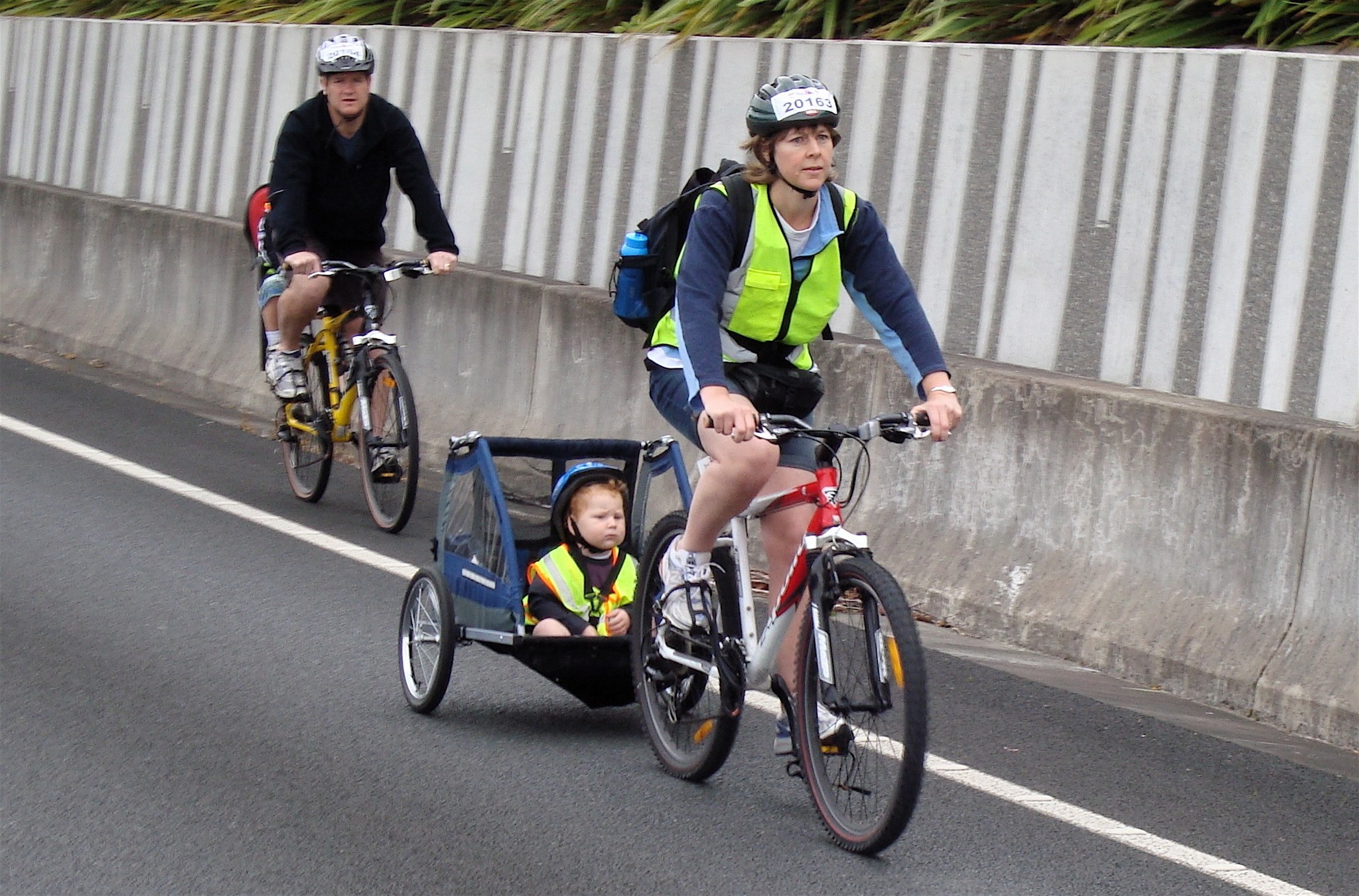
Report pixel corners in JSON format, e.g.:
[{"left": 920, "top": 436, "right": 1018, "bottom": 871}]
[{"left": 925, "top": 385, "right": 957, "bottom": 396}]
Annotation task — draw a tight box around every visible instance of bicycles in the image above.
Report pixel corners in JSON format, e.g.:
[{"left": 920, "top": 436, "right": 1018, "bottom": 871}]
[
  {"left": 629, "top": 416, "right": 931, "bottom": 855},
  {"left": 271, "top": 261, "right": 433, "bottom": 535}
]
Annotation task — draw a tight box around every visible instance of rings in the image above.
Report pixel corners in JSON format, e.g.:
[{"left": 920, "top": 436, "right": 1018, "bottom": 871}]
[{"left": 444, "top": 264, "right": 452, "bottom": 267}]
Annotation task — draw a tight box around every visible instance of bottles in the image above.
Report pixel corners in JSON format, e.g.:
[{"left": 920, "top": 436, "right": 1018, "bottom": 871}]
[{"left": 613, "top": 230, "right": 648, "bottom": 319}]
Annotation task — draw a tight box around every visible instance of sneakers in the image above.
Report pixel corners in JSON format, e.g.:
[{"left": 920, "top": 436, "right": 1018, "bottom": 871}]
[
  {"left": 264, "top": 343, "right": 281, "bottom": 386},
  {"left": 773, "top": 699, "right": 854, "bottom": 755},
  {"left": 367, "top": 443, "right": 400, "bottom": 472},
  {"left": 272, "top": 348, "right": 308, "bottom": 398},
  {"left": 659, "top": 533, "right": 726, "bottom": 630}
]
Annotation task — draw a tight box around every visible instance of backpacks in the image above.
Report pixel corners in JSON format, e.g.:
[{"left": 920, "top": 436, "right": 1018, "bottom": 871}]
[{"left": 609, "top": 158, "right": 845, "bottom": 340}]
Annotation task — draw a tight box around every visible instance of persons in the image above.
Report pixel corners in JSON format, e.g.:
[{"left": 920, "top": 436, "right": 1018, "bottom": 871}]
[
  {"left": 257, "top": 34, "right": 459, "bottom": 483},
  {"left": 608, "top": 72, "right": 965, "bottom": 756},
  {"left": 522, "top": 461, "right": 640, "bottom": 637}
]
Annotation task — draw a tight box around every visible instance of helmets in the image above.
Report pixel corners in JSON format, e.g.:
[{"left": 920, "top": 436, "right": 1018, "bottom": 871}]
[
  {"left": 549, "top": 462, "right": 626, "bottom": 542},
  {"left": 315, "top": 35, "right": 375, "bottom": 76},
  {"left": 746, "top": 73, "right": 840, "bottom": 133}
]
